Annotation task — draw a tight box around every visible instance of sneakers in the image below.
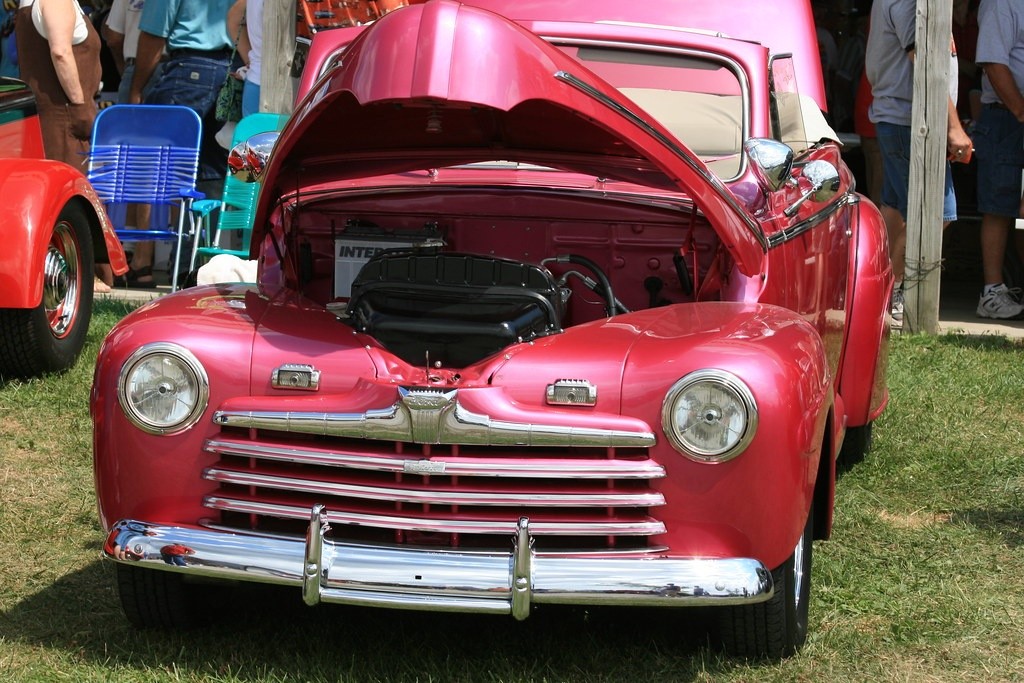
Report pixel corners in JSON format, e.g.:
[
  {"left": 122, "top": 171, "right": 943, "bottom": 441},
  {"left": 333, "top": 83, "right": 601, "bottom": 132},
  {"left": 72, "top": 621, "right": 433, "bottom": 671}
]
[{"left": 976, "top": 284, "right": 1024, "bottom": 318}]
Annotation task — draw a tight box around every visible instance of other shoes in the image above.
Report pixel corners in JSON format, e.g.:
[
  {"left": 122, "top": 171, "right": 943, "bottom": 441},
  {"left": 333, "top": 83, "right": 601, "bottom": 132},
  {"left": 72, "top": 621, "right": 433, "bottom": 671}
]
[
  {"left": 93, "top": 278, "right": 111, "bottom": 293},
  {"left": 891, "top": 283, "right": 903, "bottom": 320}
]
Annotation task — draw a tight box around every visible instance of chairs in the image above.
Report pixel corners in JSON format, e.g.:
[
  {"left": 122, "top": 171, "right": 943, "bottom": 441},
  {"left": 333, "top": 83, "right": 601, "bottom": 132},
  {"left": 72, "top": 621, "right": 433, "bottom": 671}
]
[
  {"left": 188, "top": 113, "right": 291, "bottom": 273},
  {"left": 77, "top": 104, "right": 205, "bottom": 294}
]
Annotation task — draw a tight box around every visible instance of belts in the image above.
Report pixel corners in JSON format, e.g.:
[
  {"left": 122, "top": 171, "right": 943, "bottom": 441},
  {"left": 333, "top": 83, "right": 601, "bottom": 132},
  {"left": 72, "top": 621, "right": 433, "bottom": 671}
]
[
  {"left": 169, "top": 48, "right": 229, "bottom": 60},
  {"left": 124, "top": 57, "right": 135, "bottom": 66}
]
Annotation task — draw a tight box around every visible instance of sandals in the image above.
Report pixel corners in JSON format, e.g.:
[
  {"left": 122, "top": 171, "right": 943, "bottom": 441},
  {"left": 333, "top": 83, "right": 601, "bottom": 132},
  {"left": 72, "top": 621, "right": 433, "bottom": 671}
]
[{"left": 113, "top": 266, "right": 156, "bottom": 288}]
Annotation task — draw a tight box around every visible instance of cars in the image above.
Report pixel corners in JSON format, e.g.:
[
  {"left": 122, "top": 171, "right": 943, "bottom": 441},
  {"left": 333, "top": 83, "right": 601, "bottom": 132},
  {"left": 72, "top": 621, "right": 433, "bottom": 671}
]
[
  {"left": 0, "top": 77, "right": 130, "bottom": 385},
  {"left": 89, "top": 0, "right": 896, "bottom": 663}
]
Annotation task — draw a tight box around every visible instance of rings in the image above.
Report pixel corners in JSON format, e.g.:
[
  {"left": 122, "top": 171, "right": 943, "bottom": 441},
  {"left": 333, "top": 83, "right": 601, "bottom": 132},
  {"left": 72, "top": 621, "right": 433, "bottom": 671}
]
[{"left": 958, "top": 149, "right": 961, "bottom": 154}]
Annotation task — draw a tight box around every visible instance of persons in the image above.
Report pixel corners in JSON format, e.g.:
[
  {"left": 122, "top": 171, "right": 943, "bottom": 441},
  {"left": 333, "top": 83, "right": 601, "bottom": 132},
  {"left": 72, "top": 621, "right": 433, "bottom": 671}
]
[
  {"left": 0, "top": 0, "right": 264, "bottom": 292},
  {"left": 816, "top": 0, "right": 1024, "bottom": 330}
]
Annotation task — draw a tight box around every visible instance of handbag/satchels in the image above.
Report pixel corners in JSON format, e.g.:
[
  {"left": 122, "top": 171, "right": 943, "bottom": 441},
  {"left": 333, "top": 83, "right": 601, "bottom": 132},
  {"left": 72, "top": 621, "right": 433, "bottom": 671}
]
[{"left": 215, "top": 75, "right": 244, "bottom": 123}]
